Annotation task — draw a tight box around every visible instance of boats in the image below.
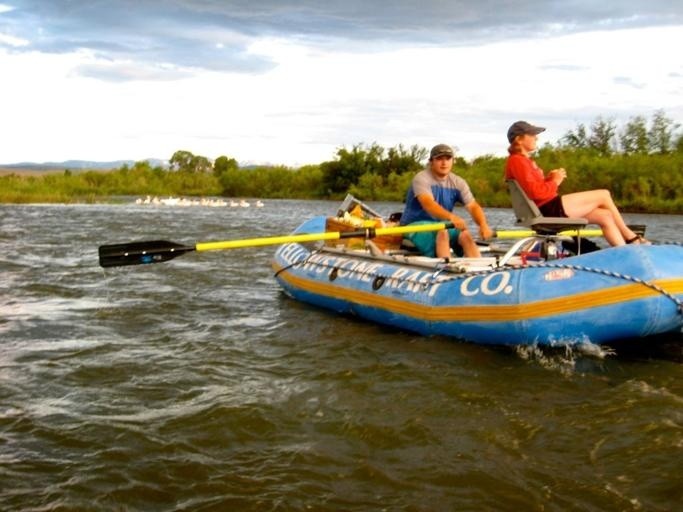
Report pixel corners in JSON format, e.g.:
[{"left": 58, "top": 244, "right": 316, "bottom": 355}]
[{"left": 270, "top": 215, "right": 682, "bottom": 351}]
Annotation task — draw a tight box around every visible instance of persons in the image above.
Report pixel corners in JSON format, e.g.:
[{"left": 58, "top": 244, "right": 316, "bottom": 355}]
[
  {"left": 503, "top": 121, "right": 651, "bottom": 248},
  {"left": 398, "top": 143, "right": 494, "bottom": 263}
]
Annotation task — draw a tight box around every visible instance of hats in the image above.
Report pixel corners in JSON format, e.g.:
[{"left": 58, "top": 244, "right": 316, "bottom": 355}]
[
  {"left": 507, "top": 121, "right": 545, "bottom": 143},
  {"left": 431, "top": 144, "right": 453, "bottom": 159}
]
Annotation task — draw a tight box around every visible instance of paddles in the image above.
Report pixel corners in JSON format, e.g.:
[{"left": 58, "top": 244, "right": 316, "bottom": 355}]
[
  {"left": 492, "top": 224, "right": 646, "bottom": 241},
  {"left": 98, "top": 223, "right": 458, "bottom": 267}
]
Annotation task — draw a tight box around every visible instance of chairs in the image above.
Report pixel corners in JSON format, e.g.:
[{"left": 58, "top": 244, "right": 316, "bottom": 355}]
[{"left": 503, "top": 177, "right": 588, "bottom": 258}]
